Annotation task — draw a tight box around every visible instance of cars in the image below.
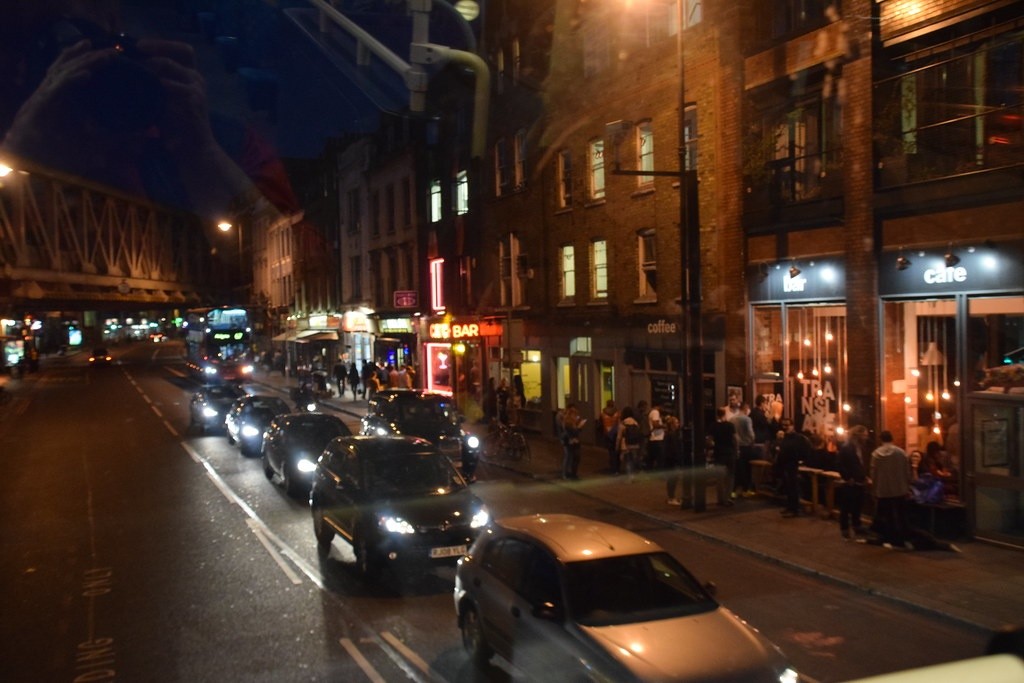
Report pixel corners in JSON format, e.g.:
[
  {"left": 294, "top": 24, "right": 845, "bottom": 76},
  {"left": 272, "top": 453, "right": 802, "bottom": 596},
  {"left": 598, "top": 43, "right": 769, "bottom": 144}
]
[
  {"left": 86, "top": 347, "right": 113, "bottom": 363},
  {"left": 189, "top": 386, "right": 249, "bottom": 438},
  {"left": 223, "top": 395, "right": 293, "bottom": 457},
  {"left": 260, "top": 412, "right": 354, "bottom": 498},
  {"left": 307, "top": 432, "right": 496, "bottom": 593},
  {"left": 452, "top": 513, "right": 820, "bottom": 683},
  {"left": 358, "top": 390, "right": 484, "bottom": 486}
]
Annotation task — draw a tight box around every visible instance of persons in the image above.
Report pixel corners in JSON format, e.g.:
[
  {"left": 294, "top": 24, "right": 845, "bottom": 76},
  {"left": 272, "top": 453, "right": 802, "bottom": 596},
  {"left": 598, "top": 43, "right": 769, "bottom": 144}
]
[
  {"left": 711, "top": 389, "right": 834, "bottom": 518},
  {"left": 869, "top": 430, "right": 913, "bottom": 550},
  {"left": 838, "top": 424, "right": 873, "bottom": 543},
  {"left": 910, "top": 441, "right": 953, "bottom": 535},
  {"left": 478, "top": 375, "right": 531, "bottom": 432},
  {"left": 360, "top": 359, "right": 420, "bottom": 413},
  {"left": 347, "top": 362, "right": 359, "bottom": 402},
  {"left": 332, "top": 358, "right": 346, "bottom": 398},
  {"left": 599, "top": 398, "right": 680, "bottom": 479},
  {"left": 554, "top": 403, "right": 589, "bottom": 480}
]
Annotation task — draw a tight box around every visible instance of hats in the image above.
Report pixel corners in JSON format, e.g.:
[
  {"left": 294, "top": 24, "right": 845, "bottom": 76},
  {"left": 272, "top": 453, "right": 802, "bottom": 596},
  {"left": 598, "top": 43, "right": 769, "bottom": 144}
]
[{"left": 928, "top": 442, "right": 945, "bottom": 452}]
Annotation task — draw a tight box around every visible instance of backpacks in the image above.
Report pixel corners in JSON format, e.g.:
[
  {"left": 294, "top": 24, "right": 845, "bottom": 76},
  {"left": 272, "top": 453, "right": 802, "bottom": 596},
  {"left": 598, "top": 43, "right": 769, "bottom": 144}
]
[{"left": 622, "top": 423, "right": 639, "bottom": 445}]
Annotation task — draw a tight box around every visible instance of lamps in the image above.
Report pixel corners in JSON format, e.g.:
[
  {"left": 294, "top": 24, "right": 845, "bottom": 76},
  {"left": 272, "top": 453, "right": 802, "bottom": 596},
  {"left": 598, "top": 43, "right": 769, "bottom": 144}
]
[{"left": 753, "top": 239, "right": 968, "bottom": 282}]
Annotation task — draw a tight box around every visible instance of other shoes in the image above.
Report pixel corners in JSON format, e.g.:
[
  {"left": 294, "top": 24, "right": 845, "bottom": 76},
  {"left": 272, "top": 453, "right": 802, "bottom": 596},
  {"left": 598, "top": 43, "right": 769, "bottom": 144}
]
[
  {"left": 780, "top": 509, "right": 795, "bottom": 517},
  {"left": 843, "top": 533, "right": 851, "bottom": 542},
  {"left": 668, "top": 497, "right": 683, "bottom": 506},
  {"left": 563, "top": 475, "right": 569, "bottom": 479}
]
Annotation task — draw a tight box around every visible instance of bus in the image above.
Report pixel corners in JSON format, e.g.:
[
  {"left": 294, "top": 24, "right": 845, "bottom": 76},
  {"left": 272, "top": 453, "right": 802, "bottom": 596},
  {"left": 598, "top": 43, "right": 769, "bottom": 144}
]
[{"left": 184, "top": 305, "right": 256, "bottom": 384}]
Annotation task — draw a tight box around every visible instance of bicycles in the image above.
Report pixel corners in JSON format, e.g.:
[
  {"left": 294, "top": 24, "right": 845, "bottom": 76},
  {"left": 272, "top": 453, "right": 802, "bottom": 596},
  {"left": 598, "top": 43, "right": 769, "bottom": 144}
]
[{"left": 481, "top": 417, "right": 532, "bottom": 462}]
